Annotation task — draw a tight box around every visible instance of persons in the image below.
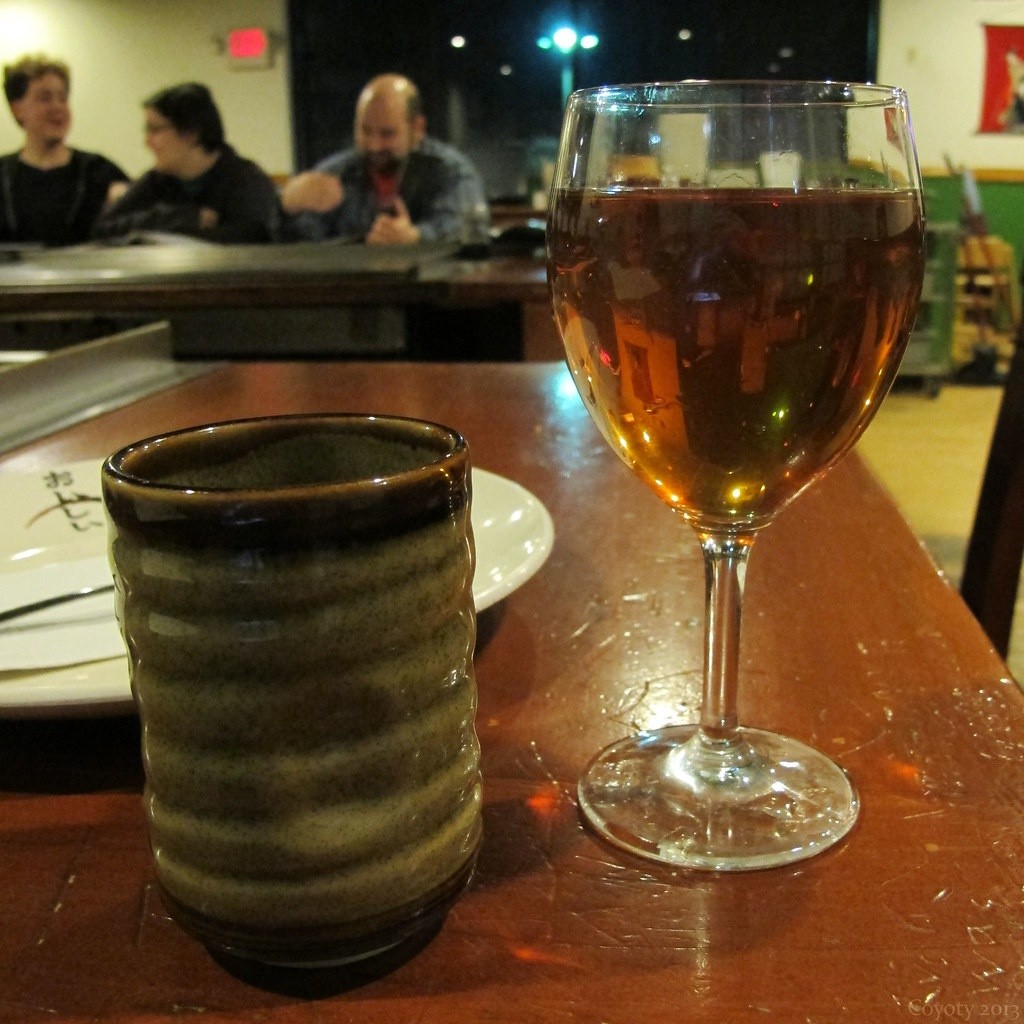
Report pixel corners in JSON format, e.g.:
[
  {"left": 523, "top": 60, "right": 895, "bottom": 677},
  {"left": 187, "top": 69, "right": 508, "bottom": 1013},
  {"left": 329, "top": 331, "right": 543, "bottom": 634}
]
[
  {"left": 0, "top": 55, "right": 135, "bottom": 247},
  {"left": 283, "top": 73, "right": 490, "bottom": 244},
  {"left": 94, "top": 82, "right": 281, "bottom": 244}
]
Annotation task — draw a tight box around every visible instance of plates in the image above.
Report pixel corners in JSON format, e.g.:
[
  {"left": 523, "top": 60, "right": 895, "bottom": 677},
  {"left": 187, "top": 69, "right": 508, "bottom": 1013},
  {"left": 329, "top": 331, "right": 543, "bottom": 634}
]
[{"left": 0, "top": 459, "right": 555, "bottom": 710}]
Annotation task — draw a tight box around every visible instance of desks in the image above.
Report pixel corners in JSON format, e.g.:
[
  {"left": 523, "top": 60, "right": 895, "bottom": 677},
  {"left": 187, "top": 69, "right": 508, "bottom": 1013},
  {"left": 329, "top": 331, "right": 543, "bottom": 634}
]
[
  {"left": 1, "top": 242, "right": 465, "bottom": 360},
  {"left": 1, "top": 361, "right": 1024, "bottom": 1021}
]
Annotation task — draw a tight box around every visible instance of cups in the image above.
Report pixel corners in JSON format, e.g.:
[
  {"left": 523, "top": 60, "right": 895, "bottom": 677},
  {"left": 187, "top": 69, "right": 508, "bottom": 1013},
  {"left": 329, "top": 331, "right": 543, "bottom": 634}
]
[{"left": 101, "top": 412, "right": 484, "bottom": 996}]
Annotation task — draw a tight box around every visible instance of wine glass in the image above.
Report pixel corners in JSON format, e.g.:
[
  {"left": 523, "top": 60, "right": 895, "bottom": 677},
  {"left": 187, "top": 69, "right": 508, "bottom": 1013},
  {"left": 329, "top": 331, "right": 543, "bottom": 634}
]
[{"left": 545, "top": 80, "right": 925, "bottom": 871}]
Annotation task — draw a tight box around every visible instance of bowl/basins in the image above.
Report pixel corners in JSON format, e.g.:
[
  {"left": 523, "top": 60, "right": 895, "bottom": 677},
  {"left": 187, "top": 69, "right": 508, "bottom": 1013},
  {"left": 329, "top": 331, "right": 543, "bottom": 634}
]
[{"left": 486, "top": 220, "right": 545, "bottom": 259}]
